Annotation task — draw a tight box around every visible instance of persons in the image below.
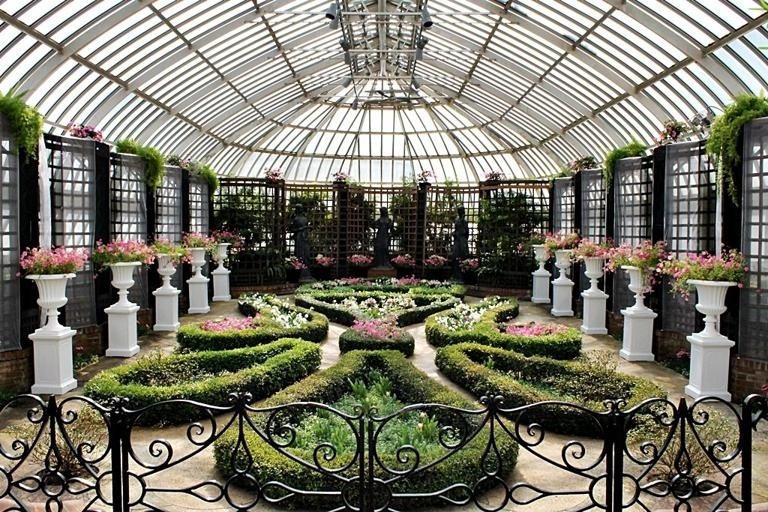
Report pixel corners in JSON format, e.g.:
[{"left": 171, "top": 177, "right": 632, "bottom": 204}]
[
  {"left": 370, "top": 206, "right": 399, "bottom": 267},
  {"left": 454, "top": 206, "right": 469, "bottom": 258},
  {"left": 292, "top": 203, "right": 314, "bottom": 257}
]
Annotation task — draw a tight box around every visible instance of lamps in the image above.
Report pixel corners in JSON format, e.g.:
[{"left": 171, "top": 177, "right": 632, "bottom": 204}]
[{"left": 325, "top": 1, "right": 433, "bottom": 112}]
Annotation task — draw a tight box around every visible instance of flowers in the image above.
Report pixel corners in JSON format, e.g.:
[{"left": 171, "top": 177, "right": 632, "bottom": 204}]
[
  {"left": 570, "top": 155, "right": 595, "bottom": 173},
  {"left": 266, "top": 165, "right": 285, "bottom": 183},
  {"left": 332, "top": 170, "right": 355, "bottom": 184},
  {"left": 482, "top": 169, "right": 506, "bottom": 181},
  {"left": 417, "top": 170, "right": 438, "bottom": 185},
  {"left": 179, "top": 155, "right": 194, "bottom": 173},
  {"left": 68, "top": 118, "right": 105, "bottom": 144},
  {"left": 659, "top": 118, "right": 685, "bottom": 146},
  {"left": 17, "top": 220, "right": 237, "bottom": 330},
  {"left": 687, "top": 106, "right": 723, "bottom": 136},
  {"left": 527, "top": 220, "right": 748, "bottom": 347}
]
